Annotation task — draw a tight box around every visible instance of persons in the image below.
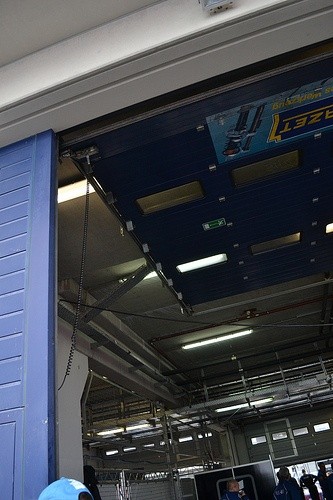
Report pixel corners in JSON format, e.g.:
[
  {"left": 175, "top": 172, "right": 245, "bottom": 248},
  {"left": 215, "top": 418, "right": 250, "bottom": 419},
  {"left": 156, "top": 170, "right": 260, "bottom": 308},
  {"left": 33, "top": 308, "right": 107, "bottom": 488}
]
[
  {"left": 83, "top": 465, "right": 102, "bottom": 500},
  {"left": 219, "top": 462, "right": 333, "bottom": 500}
]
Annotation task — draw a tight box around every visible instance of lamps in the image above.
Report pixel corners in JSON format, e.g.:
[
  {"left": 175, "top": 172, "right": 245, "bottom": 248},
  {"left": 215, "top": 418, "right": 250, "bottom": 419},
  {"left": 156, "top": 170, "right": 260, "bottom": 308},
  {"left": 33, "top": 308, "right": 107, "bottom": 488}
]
[
  {"left": 215, "top": 397, "right": 274, "bottom": 413},
  {"left": 180, "top": 328, "right": 254, "bottom": 350},
  {"left": 57, "top": 178, "right": 103, "bottom": 203}
]
